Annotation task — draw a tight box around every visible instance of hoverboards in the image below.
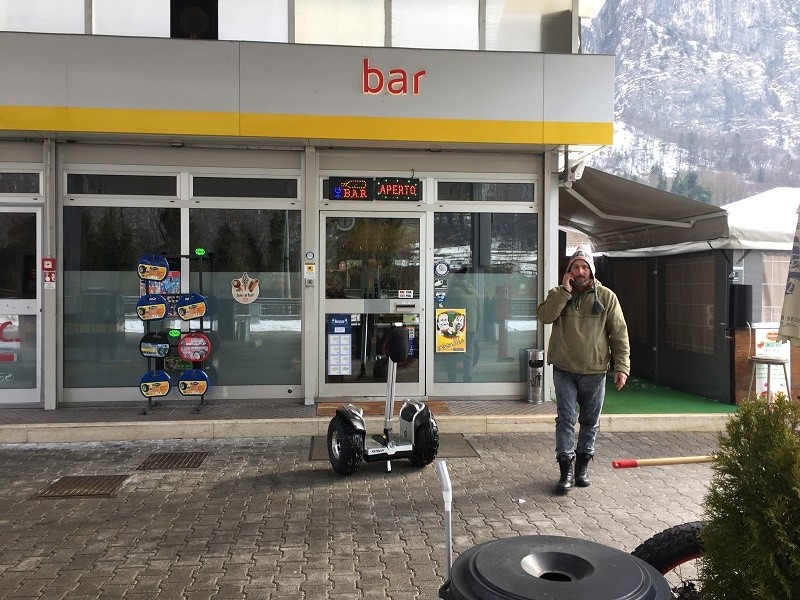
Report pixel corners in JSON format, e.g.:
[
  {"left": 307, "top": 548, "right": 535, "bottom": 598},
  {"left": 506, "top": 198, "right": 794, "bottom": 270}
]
[{"left": 327, "top": 326, "right": 440, "bottom": 477}]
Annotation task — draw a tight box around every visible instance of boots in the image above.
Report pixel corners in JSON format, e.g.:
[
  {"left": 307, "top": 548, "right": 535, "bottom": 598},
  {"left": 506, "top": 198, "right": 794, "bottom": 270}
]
[
  {"left": 573, "top": 449, "right": 594, "bottom": 487},
  {"left": 554, "top": 453, "right": 576, "bottom": 492}
]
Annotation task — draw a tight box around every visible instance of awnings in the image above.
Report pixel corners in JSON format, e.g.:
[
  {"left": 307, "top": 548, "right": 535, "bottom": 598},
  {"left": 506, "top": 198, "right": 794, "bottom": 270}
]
[{"left": 561, "top": 161, "right": 730, "bottom": 251}]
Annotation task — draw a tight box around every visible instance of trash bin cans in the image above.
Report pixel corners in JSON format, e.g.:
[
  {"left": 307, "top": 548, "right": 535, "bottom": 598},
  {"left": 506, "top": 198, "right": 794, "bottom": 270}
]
[
  {"left": 233, "top": 313, "right": 251, "bottom": 343},
  {"left": 526, "top": 349, "right": 545, "bottom": 405}
]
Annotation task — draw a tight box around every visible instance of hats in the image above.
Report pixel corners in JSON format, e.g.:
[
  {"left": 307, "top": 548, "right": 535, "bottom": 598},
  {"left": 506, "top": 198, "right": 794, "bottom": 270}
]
[{"left": 565, "top": 245, "right": 606, "bottom": 315}]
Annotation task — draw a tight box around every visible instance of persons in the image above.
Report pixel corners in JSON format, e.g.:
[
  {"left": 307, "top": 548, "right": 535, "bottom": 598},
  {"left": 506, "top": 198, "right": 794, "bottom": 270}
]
[
  {"left": 536, "top": 245, "right": 633, "bottom": 494},
  {"left": 441, "top": 267, "right": 481, "bottom": 383}
]
